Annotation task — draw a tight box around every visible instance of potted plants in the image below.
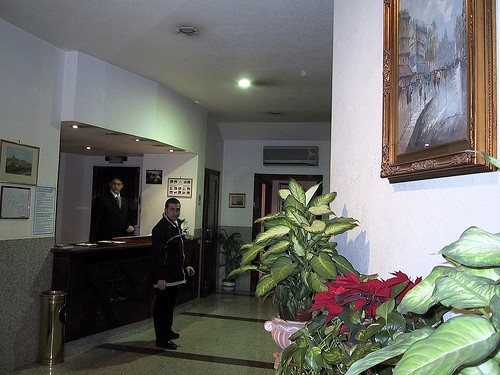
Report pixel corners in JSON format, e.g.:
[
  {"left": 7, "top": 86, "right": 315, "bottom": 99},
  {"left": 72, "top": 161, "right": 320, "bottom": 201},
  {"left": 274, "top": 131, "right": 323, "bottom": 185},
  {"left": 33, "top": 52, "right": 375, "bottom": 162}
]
[
  {"left": 226, "top": 178, "right": 360, "bottom": 375},
  {"left": 219, "top": 229, "right": 245, "bottom": 292}
]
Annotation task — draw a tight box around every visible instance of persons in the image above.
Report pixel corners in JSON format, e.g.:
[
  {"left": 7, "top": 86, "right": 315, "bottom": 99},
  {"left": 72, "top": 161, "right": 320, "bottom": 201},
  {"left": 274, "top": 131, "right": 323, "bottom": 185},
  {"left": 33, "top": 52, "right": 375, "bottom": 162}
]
[
  {"left": 152, "top": 198, "right": 196, "bottom": 349},
  {"left": 100, "top": 177, "right": 136, "bottom": 239}
]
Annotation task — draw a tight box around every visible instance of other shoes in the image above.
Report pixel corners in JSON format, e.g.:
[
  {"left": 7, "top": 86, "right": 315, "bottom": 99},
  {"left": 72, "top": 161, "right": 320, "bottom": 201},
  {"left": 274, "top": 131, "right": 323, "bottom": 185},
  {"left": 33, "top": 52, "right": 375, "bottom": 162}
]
[
  {"left": 156, "top": 338, "right": 177, "bottom": 349},
  {"left": 166, "top": 329, "right": 180, "bottom": 339}
]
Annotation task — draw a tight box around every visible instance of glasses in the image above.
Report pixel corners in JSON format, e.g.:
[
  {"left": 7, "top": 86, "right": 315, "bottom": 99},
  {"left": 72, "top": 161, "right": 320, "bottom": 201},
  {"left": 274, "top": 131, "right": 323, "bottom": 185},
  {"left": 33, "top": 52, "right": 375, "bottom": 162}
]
[{"left": 112, "top": 182, "right": 121, "bottom": 186}]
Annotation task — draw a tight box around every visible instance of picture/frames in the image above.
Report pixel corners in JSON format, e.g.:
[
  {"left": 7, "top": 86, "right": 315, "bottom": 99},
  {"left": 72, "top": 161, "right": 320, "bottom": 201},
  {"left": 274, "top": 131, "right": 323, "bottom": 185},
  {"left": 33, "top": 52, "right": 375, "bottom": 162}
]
[
  {"left": 229, "top": 192, "right": 246, "bottom": 208},
  {"left": 167, "top": 177, "right": 193, "bottom": 198},
  {"left": 0, "top": 185, "right": 31, "bottom": 219},
  {"left": 380, "top": 0, "right": 498, "bottom": 184},
  {"left": 0, "top": 139, "right": 40, "bottom": 186},
  {"left": 146, "top": 169, "right": 162, "bottom": 184}
]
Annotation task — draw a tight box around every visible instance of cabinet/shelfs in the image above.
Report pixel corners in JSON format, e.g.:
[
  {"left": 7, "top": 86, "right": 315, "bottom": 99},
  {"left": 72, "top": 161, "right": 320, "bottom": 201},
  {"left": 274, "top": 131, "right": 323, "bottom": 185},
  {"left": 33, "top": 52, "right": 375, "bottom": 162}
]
[{"left": 50, "top": 243, "right": 200, "bottom": 343}]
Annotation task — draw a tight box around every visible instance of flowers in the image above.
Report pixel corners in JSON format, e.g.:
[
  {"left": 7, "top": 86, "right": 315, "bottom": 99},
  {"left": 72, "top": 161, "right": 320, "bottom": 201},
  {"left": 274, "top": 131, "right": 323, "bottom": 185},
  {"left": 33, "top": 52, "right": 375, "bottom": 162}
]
[{"left": 278, "top": 270, "right": 452, "bottom": 375}]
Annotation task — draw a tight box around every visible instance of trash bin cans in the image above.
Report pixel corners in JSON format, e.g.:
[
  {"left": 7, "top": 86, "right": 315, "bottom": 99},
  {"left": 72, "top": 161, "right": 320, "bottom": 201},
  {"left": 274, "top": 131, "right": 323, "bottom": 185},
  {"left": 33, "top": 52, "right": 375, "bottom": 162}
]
[{"left": 39, "top": 291, "right": 68, "bottom": 365}]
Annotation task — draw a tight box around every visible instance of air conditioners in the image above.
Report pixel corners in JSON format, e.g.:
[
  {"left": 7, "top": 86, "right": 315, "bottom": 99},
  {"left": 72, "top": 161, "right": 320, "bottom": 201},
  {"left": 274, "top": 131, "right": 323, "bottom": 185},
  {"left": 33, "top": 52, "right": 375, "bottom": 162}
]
[{"left": 263, "top": 145, "right": 319, "bottom": 166}]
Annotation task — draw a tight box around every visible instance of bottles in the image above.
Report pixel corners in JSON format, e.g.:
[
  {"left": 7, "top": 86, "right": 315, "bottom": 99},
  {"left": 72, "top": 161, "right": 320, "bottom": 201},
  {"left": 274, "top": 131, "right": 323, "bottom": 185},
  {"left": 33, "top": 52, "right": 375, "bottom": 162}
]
[{"left": 182, "top": 222, "right": 189, "bottom": 235}]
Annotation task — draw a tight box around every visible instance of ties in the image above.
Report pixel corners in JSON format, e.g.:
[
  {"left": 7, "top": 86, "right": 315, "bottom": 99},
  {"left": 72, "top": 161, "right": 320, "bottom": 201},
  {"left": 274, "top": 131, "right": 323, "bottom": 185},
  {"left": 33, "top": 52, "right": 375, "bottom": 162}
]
[{"left": 115, "top": 197, "right": 119, "bottom": 209}]
